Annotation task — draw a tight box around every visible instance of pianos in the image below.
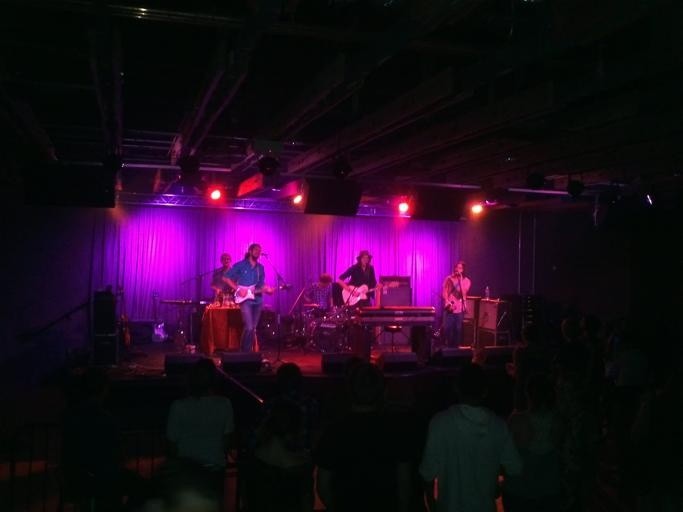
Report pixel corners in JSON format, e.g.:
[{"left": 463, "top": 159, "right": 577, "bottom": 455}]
[{"left": 361, "top": 306, "right": 435, "bottom": 323}]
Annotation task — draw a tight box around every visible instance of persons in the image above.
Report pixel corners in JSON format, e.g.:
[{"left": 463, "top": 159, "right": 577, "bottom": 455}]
[
  {"left": 336, "top": 255, "right": 383, "bottom": 305},
  {"left": 303, "top": 273, "right": 332, "bottom": 316},
  {"left": 210, "top": 244, "right": 264, "bottom": 352},
  {"left": 442, "top": 262, "right": 471, "bottom": 344}
]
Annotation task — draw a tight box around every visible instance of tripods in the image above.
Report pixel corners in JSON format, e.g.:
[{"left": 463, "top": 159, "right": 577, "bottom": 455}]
[{"left": 265, "top": 256, "right": 289, "bottom": 367}]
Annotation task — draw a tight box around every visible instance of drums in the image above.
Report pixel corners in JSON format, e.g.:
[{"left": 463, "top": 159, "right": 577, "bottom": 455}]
[{"left": 306, "top": 318, "right": 344, "bottom": 353}]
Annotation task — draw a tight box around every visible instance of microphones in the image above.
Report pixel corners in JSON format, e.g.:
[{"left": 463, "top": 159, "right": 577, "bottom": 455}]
[{"left": 261, "top": 253, "right": 268, "bottom": 256}]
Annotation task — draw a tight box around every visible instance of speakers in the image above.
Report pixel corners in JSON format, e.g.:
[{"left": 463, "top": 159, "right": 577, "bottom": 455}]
[
  {"left": 223, "top": 352, "right": 262, "bottom": 375},
  {"left": 301, "top": 176, "right": 361, "bottom": 215},
  {"left": 376, "top": 352, "right": 417, "bottom": 369},
  {"left": 23, "top": 151, "right": 122, "bottom": 207},
  {"left": 165, "top": 354, "right": 216, "bottom": 385},
  {"left": 93, "top": 291, "right": 115, "bottom": 334},
  {"left": 430, "top": 348, "right": 473, "bottom": 368},
  {"left": 321, "top": 353, "right": 355, "bottom": 374},
  {"left": 475, "top": 347, "right": 513, "bottom": 366}
]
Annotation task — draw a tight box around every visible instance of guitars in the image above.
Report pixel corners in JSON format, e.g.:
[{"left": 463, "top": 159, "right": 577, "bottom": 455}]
[
  {"left": 119, "top": 289, "right": 130, "bottom": 348},
  {"left": 234, "top": 284, "right": 292, "bottom": 303},
  {"left": 342, "top": 281, "right": 399, "bottom": 306},
  {"left": 152, "top": 289, "right": 167, "bottom": 342}
]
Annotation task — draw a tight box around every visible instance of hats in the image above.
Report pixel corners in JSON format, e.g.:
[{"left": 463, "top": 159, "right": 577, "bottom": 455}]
[{"left": 357, "top": 250, "right": 371, "bottom": 262}]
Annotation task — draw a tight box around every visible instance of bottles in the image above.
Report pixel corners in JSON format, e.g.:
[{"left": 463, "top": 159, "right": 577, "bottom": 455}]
[{"left": 484, "top": 285, "right": 490, "bottom": 301}]
